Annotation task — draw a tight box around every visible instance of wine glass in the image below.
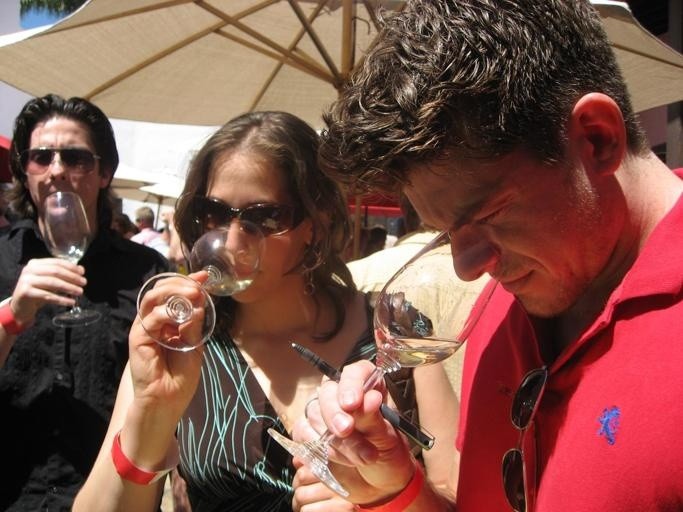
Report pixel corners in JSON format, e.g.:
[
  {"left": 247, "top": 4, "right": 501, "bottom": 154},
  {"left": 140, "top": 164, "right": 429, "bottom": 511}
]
[
  {"left": 136, "top": 218, "right": 264, "bottom": 354},
  {"left": 40, "top": 190, "right": 102, "bottom": 330},
  {"left": 265, "top": 226, "right": 516, "bottom": 497}
]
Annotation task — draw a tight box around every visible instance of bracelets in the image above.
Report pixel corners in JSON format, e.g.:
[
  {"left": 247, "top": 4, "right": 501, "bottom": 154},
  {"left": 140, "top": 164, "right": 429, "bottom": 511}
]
[
  {"left": 108, "top": 426, "right": 181, "bottom": 486},
  {"left": 351, "top": 454, "right": 425, "bottom": 511},
  {"left": 0, "top": 295, "right": 38, "bottom": 336}
]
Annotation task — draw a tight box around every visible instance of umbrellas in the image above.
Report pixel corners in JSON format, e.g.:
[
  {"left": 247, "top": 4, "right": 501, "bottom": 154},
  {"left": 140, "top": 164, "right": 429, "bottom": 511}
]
[
  {"left": 99, "top": 164, "right": 185, "bottom": 223},
  {"left": 0, "top": 0, "right": 682, "bottom": 263}
]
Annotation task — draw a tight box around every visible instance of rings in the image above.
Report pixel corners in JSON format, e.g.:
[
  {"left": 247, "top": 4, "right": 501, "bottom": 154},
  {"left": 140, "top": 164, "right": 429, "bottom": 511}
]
[{"left": 303, "top": 392, "right": 319, "bottom": 420}]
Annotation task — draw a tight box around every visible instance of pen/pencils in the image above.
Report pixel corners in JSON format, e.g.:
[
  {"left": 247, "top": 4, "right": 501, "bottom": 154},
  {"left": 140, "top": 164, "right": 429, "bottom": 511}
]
[{"left": 288, "top": 340, "right": 435, "bottom": 450}]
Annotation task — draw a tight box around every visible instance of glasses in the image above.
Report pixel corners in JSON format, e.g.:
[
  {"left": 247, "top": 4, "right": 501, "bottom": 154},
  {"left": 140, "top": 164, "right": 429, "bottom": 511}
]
[
  {"left": 500, "top": 366, "right": 550, "bottom": 512},
  {"left": 15, "top": 147, "right": 102, "bottom": 176},
  {"left": 193, "top": 196, "right": 308, "bottom": 238}
]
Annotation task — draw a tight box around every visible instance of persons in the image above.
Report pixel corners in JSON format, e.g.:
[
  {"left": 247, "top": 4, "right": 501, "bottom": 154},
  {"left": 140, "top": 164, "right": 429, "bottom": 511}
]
[
  {"left": 330, "top": 186, "right": 495, "bottom": 398},
  {"left": 105, "top": 205, "right": 191, "bottom": 274},
  {"left": 288, "top": 0, "right": 683, "bottom": 509},
  {"left": 0, "top": 92, "right": 179, "bottom": 511},
  {"left": 72, "top": 109, "right": 463, "bottom": 511}
]
